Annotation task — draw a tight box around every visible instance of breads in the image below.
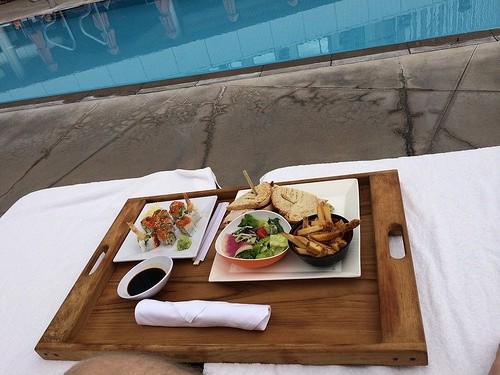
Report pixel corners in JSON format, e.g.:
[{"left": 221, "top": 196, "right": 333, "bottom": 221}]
[
  {"left": 226, "top": 181, "right": 274, "bottom": 211},
  {"left": 271, "top": 186, "right": 330, "bottom": 223}
]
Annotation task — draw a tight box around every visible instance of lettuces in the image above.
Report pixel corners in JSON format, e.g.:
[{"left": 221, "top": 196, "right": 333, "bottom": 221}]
[{"left": 223, "top": 213, "right": 289, "bottom": 259}]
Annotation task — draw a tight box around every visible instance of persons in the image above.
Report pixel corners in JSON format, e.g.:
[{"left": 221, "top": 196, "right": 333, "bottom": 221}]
[
  {"left": 11, "top": 13, "right": 55, "bottom": 30},
  {"left": 62, "top": 342, "right": 499, "bottom": 375}
]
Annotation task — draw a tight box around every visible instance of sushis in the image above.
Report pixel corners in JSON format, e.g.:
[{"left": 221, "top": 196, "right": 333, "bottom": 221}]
[{"left": 125, "top": 192, "right": 201, "bottom": 251}]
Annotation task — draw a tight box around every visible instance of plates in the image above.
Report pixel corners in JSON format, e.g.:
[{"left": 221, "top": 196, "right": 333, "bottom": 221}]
[
  {"left": 112, "top": 195, "right": 218, "bottom": 263},
  {"left": 208, "top": 178, "right": 361, "bottom": 282}
]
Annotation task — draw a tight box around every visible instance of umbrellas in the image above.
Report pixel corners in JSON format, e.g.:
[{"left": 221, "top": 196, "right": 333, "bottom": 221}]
[{"left": 29, "top": 1, "right": 306, "bottom": 76}]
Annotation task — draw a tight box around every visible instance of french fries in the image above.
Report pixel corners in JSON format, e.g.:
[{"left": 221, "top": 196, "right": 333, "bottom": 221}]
[{"left": 281, "top": 202, "right": 362, "bottom": 257}]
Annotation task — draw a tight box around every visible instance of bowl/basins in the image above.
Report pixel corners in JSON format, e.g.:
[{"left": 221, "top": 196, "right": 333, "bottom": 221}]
[
  {"left": 288, "top": 214, "right": 353, "bottom": 267},
  {"left": 215, "top": 211, "right": 292, "bottom": 268},
  {"left": 117, "top": 255, "right": 173, "bottom": 300}
]
